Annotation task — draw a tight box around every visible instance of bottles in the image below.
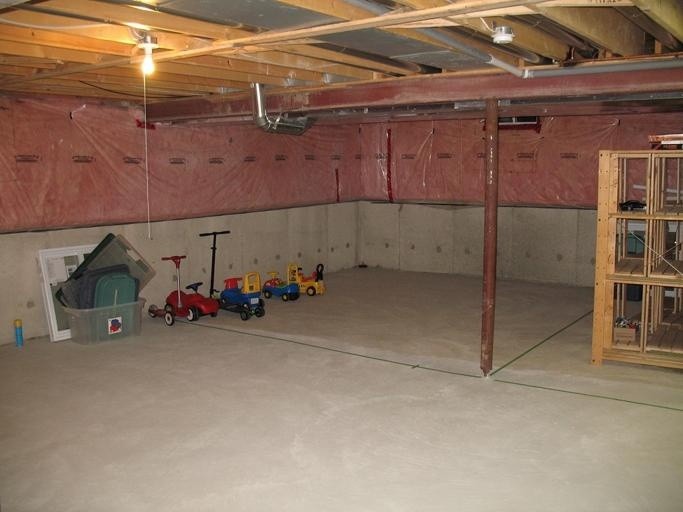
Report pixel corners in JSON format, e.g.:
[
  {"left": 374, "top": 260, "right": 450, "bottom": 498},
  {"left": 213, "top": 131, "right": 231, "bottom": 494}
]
[{"left": 12, "top": 318, "right": 24, "bottom": 349}]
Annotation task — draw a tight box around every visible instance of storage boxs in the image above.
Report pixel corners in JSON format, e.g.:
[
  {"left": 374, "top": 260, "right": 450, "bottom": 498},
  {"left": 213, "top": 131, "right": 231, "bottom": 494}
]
[
  {"left": 611, "top": 321, "right": 639, "bottom": 342},
  {"left": 60, "top": 297, "right": 146, "bottom": 346}
]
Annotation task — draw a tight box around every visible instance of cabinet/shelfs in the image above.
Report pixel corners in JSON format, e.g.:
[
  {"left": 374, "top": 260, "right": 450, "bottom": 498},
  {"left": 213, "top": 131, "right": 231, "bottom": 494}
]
[{"left": 589, "top": 142, "right": 682, "bottom": 368}]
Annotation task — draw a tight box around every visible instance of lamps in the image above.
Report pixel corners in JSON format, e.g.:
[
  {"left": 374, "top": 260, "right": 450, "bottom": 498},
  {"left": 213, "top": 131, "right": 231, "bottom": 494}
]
[{"left": 136, "top": 36, "right": 160, "bottom": 77}]
